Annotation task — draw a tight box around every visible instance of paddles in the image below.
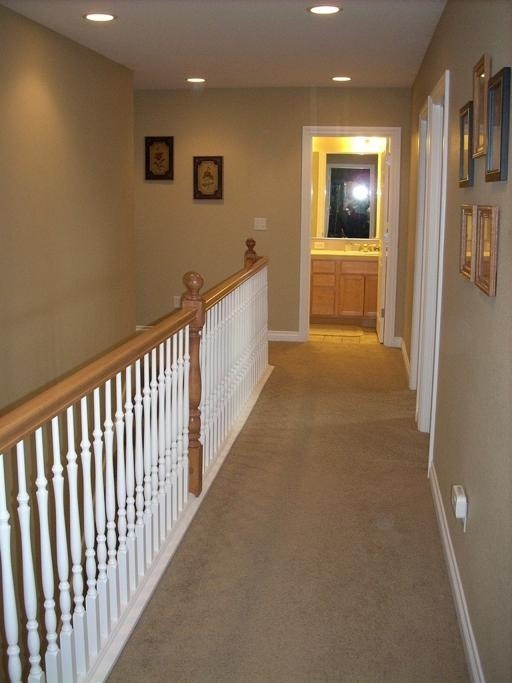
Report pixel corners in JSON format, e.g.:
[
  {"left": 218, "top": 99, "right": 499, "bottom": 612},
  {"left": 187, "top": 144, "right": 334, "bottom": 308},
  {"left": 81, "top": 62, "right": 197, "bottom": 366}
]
[{"left": 310, "top": 323, "right": 364, "bottom": 336}]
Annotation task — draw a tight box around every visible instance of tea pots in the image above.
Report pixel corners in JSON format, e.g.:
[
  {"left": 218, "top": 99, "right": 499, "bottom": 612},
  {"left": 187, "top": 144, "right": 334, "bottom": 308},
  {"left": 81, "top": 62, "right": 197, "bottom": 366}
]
[{"left": 316, "top": 153, "right": 380, "bottom": 238}]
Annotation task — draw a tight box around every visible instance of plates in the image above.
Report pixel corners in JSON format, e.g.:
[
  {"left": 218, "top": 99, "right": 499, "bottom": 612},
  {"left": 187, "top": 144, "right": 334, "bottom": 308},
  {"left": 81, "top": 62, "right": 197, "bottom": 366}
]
[{"left": 311, "top": 259, "right": 377, "bottom": 319}]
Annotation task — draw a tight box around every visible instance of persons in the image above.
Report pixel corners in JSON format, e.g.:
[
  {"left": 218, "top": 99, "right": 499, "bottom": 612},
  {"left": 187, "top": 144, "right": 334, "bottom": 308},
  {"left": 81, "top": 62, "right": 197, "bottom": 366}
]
[{"left": 345, "top": 194, "right": 370, "bottom": 222}]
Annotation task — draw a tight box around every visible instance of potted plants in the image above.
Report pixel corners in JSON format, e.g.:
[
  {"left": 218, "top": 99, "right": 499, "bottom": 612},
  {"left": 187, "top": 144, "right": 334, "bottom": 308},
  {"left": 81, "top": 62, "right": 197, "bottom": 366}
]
[
  {"left": 144, "top": 135, "right": 224, "bottom": 199},
  {"left": 456, "top": 48, "right": 511, "bottom": 298}
]
